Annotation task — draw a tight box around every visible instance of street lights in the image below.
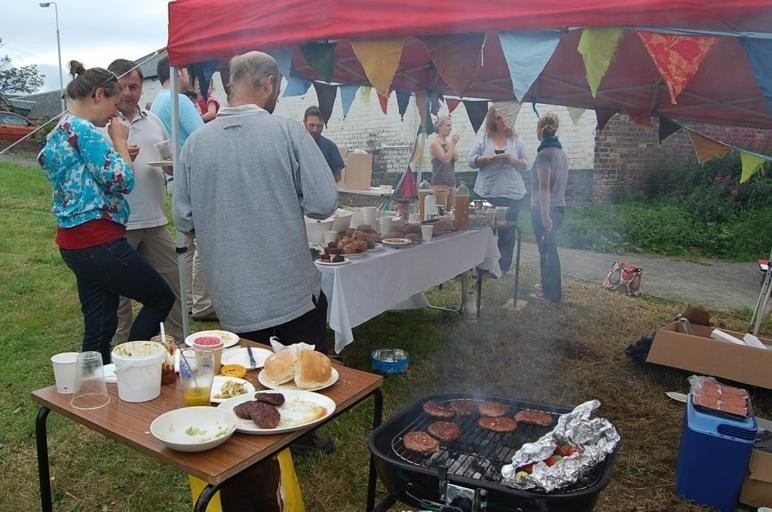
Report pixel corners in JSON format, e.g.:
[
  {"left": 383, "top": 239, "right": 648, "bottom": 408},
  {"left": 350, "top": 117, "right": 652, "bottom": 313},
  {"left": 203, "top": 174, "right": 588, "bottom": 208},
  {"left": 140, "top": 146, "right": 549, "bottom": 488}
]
[{"left": 39, "top": 2, "right": 66, "bottom": 112}]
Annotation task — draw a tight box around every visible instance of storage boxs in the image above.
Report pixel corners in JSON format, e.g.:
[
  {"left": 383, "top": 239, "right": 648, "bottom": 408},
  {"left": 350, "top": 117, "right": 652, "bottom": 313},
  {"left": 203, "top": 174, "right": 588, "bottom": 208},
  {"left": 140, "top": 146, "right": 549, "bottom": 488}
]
[
  {"left": 337, "top": 148, "right": 373, "bottom": 190},
  {"left": 740, "top": 449, "right": 771, "bottom": 509},
  {"left": 673, "top": 391, "right": 756, "bottom": 511},
  {"left": 647, "top": 320, "right": 772, "bottom": 391}
]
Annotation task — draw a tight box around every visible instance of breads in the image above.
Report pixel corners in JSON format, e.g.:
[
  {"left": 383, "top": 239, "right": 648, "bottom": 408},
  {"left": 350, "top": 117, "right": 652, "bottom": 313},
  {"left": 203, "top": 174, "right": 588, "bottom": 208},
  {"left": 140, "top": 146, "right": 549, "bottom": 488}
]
[
  {"left": 264, "top": 351, "right": 294, "bottom": 386},
  {"left": 294, "top": 349, "right": 332, "bottom": 388}
]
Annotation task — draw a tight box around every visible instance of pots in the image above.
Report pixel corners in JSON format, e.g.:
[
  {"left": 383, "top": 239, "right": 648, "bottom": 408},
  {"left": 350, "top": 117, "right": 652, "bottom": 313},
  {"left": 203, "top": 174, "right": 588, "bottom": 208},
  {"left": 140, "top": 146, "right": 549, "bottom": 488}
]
[{"left": 468, "top": 198, "right": 494, "bottom": 210}]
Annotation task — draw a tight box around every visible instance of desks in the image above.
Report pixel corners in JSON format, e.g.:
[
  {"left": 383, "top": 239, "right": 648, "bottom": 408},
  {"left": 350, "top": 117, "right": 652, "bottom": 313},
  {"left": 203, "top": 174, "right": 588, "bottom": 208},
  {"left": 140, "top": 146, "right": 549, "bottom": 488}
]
[
  {"left": 310, "top": 225, "right": 503, "bottom": 352},
  {"left": 337, "top": 187, "right": 429, "bottom": 211},
  {"left": 439, "top": 219, "right": 520, "bottom": 315},
  {"left": 28, "top": 333, "right": 384, "bottom": 512}
]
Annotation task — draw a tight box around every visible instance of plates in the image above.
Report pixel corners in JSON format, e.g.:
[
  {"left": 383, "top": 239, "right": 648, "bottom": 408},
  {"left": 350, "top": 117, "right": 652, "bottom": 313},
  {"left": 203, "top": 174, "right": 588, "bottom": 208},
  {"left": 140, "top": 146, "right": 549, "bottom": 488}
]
[
  {"left": 381, "top": 238, "right": 413, "bottom": 245},
  {"left": 192, "top": 335, "right": 223, "bottom": 348},
  {"left": 145, "top": 162, "right": 173, "bottom": 169},
  {"left": 176, "top": 347, "right": 185, "bottom": 372},
  {"left": 256, "top": 366, "right": 339, "bottom": 391},
  {"left": 316, "top": 256, "right": 349, "bottom": 265},
  {"left": 221, "top": 348, "right": 274, "bottom": 370},
  {"left": 185, "top": 329, "right": 241, "bottom": 349},
  {"left": 215, "top": 387, "right": 337, "bottom": 436},
  {"left": 193, "top": 375, "right": 255, "bottom": 404},
  {"left": 94, "top": 362, "right": 118, "bottom": 384},
  {"left": 327, "top": 242, "right": 387, "bottom": 259}
]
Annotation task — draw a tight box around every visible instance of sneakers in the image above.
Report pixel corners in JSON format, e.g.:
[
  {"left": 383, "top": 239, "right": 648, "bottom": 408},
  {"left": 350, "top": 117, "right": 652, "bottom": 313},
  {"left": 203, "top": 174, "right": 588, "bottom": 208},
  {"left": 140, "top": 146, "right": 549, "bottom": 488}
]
[{"left": 529, "top": 284, "right": 545, "bottom": 301}]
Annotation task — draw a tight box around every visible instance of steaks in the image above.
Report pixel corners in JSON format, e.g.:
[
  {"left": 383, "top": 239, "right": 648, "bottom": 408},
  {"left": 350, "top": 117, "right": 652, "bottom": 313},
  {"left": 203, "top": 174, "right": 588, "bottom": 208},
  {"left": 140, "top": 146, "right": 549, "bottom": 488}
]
[
  {"left": 233, "top": 393, "right": 285, "bottom": 429},
  {"left": 403, "top": 399, "right": 554, "bottom": 454}
]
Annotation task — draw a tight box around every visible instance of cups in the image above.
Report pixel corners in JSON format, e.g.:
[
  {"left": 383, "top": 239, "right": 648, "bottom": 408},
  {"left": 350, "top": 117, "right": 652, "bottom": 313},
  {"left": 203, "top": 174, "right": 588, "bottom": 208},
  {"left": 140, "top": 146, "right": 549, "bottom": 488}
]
[
  {"left": 178, "top": 347, "right": 215, "bottom": 404},
  {"left": 363, "top": 207, "right": 377, "bottom": 228},
  {"left": 493, "top": 145, "right": 506, "bottom": 155},
  {"left": 486, "top": 209, "right": 495, "bottom": 226},
  {"left": 324, "top": 230, "right": 338, "bottom": 246},
  {"left": 153, "top": 140, "right": 173, "bottom": 160},
  {"left": 420, "top": 225, "right": 433, "bottom": 240},
  {"left": 380, "top": 184, "right": 393, "bottom": 192},
  {"left": 50, "top": 352, "right": 82, "bottom": 395},
  {"left": 496, "top": 207, "right": 508, "bottom": 224},
  {"left": 436, "top": 205, "right": 445, "bottom": 216},
  {"left": 70, "top": 350, "right": 112, "bottom": 411},
  {"left": 379, "top": 218, "right": 393, "bottom": 236}
]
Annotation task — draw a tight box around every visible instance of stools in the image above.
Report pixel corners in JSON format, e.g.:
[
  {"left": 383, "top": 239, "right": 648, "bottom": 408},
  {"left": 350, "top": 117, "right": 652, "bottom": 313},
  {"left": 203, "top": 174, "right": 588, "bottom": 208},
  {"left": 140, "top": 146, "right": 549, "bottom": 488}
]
[{"left": 756, "top": 258, "right": 772, "bottom": 296}]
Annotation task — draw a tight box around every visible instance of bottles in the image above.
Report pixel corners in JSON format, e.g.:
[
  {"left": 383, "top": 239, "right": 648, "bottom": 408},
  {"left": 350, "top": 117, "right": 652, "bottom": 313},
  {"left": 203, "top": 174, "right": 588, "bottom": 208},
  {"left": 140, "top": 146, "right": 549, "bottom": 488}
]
[
  {"left": 424, "top": 192, "right": 436, "bottom": 221},
  {"left": 397, "top": 197, "right": 409, "bottom": 219},
  {"left": 418, "top": 177, "right": 432, "bottom": 211},
  {"left": 408, "top": 204, "right": 417, "bottom": 225},
  {"left": 456, "top": 178, "right": 470, "bottom": 231},
  {"left": 676, "top": 312, "right": 695, "bottom": 336},
  {"left": 152, "top": 335, "right": 176, "bottom": 385}
]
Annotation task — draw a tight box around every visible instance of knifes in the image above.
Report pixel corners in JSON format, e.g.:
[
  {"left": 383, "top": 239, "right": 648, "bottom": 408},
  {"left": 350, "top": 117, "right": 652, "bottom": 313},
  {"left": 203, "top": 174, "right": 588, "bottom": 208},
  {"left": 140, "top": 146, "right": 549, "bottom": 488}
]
[{"left": 247, "top": 343, "right": 258, "bottom": 366}]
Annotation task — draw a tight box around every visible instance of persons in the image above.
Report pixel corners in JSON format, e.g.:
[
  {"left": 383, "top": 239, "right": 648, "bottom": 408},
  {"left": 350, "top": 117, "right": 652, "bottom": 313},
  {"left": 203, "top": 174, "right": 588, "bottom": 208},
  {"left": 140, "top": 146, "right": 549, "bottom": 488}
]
[
  {"left": 32, "top": 60, "right": 175, "bottom": 372},
  {"left": 192, "top": 70, "right": 222, "bottom": 122},
  {"left": 302, "top": 106, "right": 347, "bottom": 183},
  {"left": 426, "top": 114, "right": 460, "bottom": 210},
  {"left": 85, "top": 57, "right": 184, "bottom": 353},
  {"left": 523, "top": 110, "right": 570, "bottom": 303},
  {"left": 151, "top": 55, "right": 222, "bottom": 323},
  {"left": 466, "top": 104, "right": 531, "bottom": 279},
  {"left": 170, "top": 49, "right": 340, "bottom": 459}
]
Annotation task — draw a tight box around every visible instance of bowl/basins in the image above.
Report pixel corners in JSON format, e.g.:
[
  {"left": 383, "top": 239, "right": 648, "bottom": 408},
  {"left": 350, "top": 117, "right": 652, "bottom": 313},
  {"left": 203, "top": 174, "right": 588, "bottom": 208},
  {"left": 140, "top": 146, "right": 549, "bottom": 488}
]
[
  {"left": 371, "top": 348, "right": 411, "bottom": 375},
  {"left": 150, "top": 406, "right": 240, "bottom": 453}
]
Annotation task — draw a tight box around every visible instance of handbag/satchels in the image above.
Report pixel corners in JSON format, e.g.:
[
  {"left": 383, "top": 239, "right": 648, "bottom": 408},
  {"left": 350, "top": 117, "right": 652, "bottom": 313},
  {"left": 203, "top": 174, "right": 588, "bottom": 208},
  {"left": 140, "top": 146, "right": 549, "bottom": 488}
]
[
  {"left": 632, "top": 336, "right": 681, "bottom": 375},
  {"left": 603, "top": 261, "right": 643, "bottom": 296}
]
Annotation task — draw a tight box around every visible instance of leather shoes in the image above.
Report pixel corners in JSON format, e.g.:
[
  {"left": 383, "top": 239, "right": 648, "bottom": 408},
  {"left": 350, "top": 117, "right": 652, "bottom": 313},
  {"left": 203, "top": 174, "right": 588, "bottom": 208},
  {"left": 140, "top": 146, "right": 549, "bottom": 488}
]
[{"left": 291, "top": 436, "right": 335, "bottom": 456}]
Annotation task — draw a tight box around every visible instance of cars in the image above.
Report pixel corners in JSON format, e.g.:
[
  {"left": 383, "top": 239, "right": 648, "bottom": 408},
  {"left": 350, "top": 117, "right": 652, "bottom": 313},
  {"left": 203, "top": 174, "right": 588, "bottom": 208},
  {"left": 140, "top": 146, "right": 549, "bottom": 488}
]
[{"left": 0, "top": 111, "right": 45, "bottom": 143}]
